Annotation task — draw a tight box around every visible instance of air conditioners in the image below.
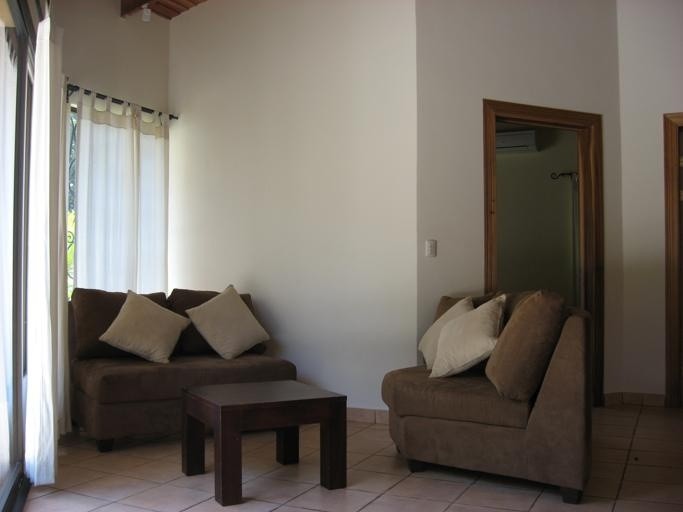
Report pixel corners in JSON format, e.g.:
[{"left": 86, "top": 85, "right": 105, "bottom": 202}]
[{"left": 494, "top": 129, "right": 540, "bottom": 154}]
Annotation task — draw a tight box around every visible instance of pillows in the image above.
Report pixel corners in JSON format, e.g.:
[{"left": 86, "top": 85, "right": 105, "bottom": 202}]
[
  {"left": 417, "top": 293, "right": 507, "bottom": 380},
  {"left": 97, "top": 283, "right": 271, "bottom": 365}
]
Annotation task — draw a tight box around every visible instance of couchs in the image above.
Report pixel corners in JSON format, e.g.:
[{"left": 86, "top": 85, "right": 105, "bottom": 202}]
[{"left": 65, "top": 286, "right": 297, "bottom": 457}]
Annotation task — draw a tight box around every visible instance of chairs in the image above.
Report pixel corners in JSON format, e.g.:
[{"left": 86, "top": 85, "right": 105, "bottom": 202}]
[{"left": 380, "top": 291, "right": 594, "bottom": 504}]
